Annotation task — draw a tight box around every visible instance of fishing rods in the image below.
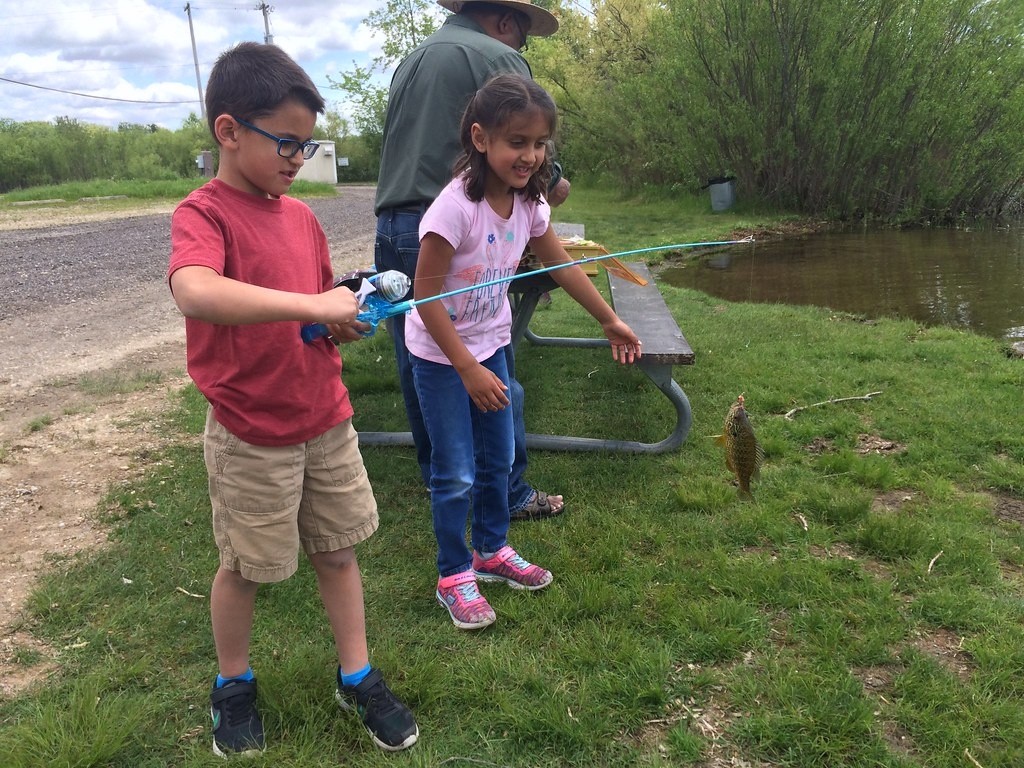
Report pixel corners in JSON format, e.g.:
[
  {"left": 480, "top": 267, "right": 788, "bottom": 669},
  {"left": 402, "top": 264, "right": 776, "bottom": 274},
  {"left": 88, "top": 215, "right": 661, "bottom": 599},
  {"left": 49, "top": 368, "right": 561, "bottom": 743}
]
[{"left": 300, "top": 235, "right": 755, "bottom": 344}]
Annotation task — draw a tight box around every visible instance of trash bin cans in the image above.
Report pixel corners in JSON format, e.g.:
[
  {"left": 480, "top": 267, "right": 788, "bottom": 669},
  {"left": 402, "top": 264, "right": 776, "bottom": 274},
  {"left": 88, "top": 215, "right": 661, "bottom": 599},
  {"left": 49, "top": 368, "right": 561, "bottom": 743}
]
[{"left": 709, "top": 179, "right": 734, "bottom": 212}]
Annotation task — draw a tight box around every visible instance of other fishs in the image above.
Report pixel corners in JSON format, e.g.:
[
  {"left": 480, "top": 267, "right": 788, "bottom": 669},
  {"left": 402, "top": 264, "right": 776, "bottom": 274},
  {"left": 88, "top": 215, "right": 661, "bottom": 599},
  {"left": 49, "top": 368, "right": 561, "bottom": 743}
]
[{"left": 724, "top": 402, "right": 766, "bottom": 503}]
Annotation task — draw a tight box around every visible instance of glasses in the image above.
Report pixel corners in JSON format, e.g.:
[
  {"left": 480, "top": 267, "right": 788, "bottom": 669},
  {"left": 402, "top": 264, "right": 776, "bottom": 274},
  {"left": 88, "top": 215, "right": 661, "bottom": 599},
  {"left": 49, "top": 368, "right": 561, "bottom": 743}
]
[
  {"left": 233, "top": 116, "right": 320, "bottom": 160},
  {"left": 512, "top": 12, "right": 529, "bottom": 55}
]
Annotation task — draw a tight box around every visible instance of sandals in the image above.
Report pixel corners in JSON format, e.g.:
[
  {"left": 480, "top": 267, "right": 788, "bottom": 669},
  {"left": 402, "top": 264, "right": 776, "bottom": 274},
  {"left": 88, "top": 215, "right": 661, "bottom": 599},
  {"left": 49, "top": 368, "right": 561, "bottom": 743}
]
[{"left": 510, "top": 490, "right": 566, "bottom": 520}]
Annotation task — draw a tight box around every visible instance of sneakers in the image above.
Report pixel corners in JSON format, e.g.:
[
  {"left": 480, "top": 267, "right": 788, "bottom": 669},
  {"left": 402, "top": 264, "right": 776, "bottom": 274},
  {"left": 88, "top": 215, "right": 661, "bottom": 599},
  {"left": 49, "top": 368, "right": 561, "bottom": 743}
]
[
  {"left": 335, "top": 663, "right": 420, "bottom": 752},
  {"left": 435, "top": 569, "right": 497, "bottom": 630},
  {"left": 472, "top": 545, "right": 554, "bottom": 591},
  {"left": 210, "top": 677, "right": 267, "bottom": 760}
]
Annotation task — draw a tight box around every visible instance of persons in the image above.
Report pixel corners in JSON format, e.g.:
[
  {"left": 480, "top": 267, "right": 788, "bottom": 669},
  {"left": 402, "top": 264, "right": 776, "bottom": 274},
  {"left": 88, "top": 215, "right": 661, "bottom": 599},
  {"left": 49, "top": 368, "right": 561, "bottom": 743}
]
[
  {"left": 373, "top": 0, "right": 643, "bottom": 631},
  {"left": 166, "top": 44, "right": 419, "bottom": 758}
]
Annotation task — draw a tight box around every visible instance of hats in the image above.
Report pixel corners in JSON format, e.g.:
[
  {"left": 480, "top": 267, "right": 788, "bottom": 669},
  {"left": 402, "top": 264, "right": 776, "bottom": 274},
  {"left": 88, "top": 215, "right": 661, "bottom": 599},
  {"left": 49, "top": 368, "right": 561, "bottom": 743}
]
[{"left": 436, "top": 0, "right": 559, "bottom": 44}]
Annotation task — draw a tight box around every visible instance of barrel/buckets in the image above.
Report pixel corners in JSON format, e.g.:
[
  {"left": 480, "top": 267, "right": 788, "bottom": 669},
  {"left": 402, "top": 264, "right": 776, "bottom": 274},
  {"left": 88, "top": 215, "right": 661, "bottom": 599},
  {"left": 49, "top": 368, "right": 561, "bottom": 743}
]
[{"left": 701, "top": 176, "right": 734, "bottom": 211}]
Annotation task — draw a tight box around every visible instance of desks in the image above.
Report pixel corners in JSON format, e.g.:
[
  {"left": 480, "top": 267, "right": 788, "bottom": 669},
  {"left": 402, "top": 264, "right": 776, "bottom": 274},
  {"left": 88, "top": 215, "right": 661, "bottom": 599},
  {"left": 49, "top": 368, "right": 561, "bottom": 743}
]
[{"left": 507, "top": 224, "right": 694, "bottom": 453}]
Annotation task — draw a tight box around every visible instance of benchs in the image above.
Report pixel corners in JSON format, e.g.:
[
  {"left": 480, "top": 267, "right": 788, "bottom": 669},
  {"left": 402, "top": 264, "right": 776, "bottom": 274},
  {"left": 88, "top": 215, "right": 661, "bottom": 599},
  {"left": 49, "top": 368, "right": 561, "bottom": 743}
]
[{"left": 604, "top": 259, "right": 696, "bottom": 453}]
